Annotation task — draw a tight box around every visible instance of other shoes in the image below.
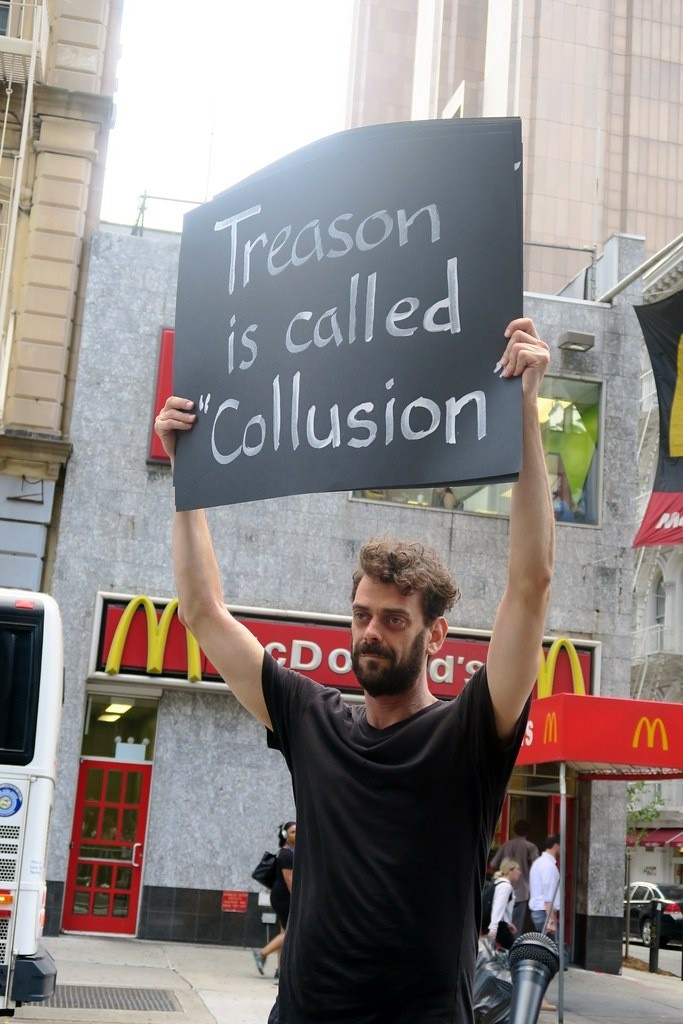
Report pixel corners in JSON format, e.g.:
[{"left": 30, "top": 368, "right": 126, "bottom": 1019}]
[
  {"left": 273, "top": 969, "right": 279, "bottom": 985},
  {"left": 253, "top": 949, "right": 266, "bottom": 976},
  {"left": 540, "top": 997, "right": 556, "bottom": 1010}
]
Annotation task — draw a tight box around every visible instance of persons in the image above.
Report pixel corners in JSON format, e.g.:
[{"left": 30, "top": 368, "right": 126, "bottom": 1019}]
[
  {"left": 488, "top": 858, "right": 520, "bottom": 938},
  {"left": 155, "top": 317, "right": 557, "bottom": 1024},
  {"left": 490, "top": 820, "right": 540, "bottom": 938},
  {"left": 251, "top": 821, "right": 295, "bottom": 977},
  {"left": 529, "top": 833, "right": 569, "bottom": 1011}
]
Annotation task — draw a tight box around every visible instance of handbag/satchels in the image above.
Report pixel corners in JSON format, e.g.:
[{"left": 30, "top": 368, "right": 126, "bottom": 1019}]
[{"left": 252, "top": 852, "right": 278, "bottom": 891}]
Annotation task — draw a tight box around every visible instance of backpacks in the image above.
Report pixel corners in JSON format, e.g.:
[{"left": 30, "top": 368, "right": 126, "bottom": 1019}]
[{"left": 480, "top": 879, "right": 514, "bottom": 935}]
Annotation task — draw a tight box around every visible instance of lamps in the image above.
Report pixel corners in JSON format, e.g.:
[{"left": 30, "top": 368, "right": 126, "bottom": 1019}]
[
  {"left": 558, "top": 332, "right": 595, "bottom": 352},
  {"left": 97, "top": 712, "right": 120, "bottom": 722},
  {"left": 105, "top": 695, "right": 133, "bottom": 715}
]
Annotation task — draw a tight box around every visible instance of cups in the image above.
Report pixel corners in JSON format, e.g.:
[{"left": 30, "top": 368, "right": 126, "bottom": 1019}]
[{"left": 418, "top": 495, "right": 425, "bottom": 504}]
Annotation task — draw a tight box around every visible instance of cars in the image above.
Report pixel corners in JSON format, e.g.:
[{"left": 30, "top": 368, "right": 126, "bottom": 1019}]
[{"left": 623, "top": 881, "right": 683, "bottom": 948}]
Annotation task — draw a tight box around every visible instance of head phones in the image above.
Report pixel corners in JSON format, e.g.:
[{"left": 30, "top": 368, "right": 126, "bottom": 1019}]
[{"left": 282, "top": 821, "right": 290, "bottom": 839}]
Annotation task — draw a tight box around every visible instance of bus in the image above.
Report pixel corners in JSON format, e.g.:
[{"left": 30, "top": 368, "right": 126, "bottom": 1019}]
[{"left": 0, "top": 585, "right": 67, "bottom": 1009}]
